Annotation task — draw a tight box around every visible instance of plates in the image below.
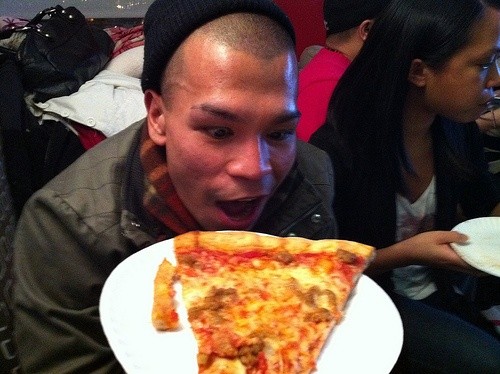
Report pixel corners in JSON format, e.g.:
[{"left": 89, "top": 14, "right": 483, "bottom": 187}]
[
  {"left": 98, "top": 229, "right": 404, "bottom": 374},
  {"left": 450, "top": 215, "right": 499, "bottom": 278}
]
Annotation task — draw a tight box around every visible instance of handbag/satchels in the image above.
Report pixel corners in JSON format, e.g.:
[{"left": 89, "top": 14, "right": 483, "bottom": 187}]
[{"left": 0, "top": 4, "right": 115, "bottom": 98}]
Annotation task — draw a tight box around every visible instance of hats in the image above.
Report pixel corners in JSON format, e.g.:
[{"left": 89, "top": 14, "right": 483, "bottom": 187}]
[
  {"left": 324, "top": 0, "right": 386, "bottom": 34},
  {"left": 141, "top": 0, "right": 296, "bottom": 94}
]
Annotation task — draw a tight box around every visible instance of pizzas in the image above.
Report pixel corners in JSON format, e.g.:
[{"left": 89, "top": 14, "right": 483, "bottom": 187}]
[
  {"left": 173, "top": 231, "right": 374, "bottom": 374},
  {"left": 151, "top": 258, "right": 180, "bottom": 330}
]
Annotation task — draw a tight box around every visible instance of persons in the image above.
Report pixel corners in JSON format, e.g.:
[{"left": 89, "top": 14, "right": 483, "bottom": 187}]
[
  {"left": 308, "top": 1, "right": 500, "bottom": 373},
  {"left": 11, "top": 1, "right": 337, "bottom": 374},
  {"left": 296, "top": 0, "right": 393, "bottom": 143},
  {"left": 475, "top": 107, "right": 500, "bottom": 135}
]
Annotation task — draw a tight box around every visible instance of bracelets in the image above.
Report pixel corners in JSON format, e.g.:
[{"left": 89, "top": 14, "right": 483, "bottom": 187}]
[{"left": 489, "top": 109, "right": 497, "bottom": 129}]
